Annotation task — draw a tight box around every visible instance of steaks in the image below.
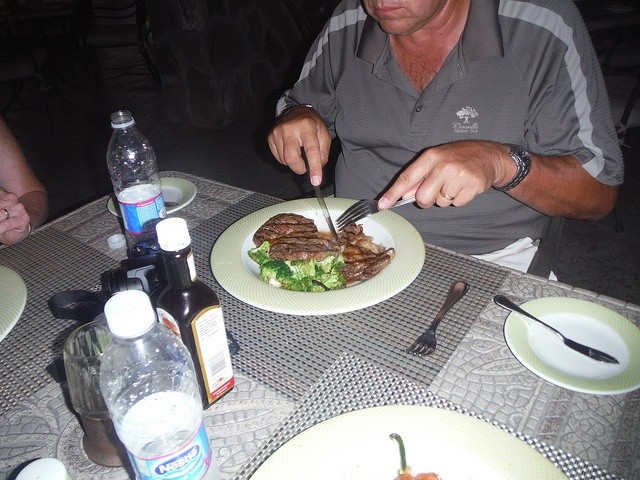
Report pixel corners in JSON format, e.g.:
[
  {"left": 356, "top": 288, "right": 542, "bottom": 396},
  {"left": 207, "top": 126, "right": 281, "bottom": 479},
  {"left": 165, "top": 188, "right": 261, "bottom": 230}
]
[{"left": 253, "top": 213, "right": 395, "bottom": 282}]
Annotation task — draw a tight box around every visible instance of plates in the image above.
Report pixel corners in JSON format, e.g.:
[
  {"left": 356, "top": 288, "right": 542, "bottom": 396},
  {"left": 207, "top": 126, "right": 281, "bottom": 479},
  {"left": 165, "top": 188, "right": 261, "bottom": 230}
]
[
  {"left": 248, "top": 403, "right": 572, "bottom": 480},
  {"left": 504, "top": 296, "right": 640, "bottom": 395},
  {"left": 108, "top": 177, "right": 197, "bottom": 217},
  {"left": 210, "top": 196, "right": 426, "bottom": 316},
  {"left": 1, "top": 265, "right": 30, "bottom": 342}
]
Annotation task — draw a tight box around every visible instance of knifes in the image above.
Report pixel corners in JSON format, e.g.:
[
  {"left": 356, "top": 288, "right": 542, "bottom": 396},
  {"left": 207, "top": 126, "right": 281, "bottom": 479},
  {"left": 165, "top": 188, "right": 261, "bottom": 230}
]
[
  {"left": 495, "top": 291, "right": 619, "bottom": 365},
  {"left": 312, "top": 183, "right": 339, "bottom": 241}
]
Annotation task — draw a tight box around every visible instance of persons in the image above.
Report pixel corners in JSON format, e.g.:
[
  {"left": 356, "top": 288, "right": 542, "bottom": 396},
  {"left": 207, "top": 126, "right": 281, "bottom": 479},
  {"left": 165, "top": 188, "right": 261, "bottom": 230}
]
[
  {"left": 0, "top": 119, "right": 49, "bottom": 246},
  {"left": 265, "top": 0, "right": 625, "bottom": 278}
]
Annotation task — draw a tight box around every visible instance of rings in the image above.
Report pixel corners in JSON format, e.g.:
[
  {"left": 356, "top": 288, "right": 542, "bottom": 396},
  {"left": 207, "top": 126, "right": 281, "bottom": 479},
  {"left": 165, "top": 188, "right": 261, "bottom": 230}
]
[
  {"left": 5, "top": 209, "right": 11, "bottom": 218},
  {"left": 440, "top": 190, "right": 453, "bottom": 199}
]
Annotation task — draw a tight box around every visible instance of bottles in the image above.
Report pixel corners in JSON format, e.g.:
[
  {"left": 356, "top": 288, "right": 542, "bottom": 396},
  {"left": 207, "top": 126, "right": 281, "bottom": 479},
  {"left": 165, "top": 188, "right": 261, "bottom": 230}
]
[
  {"left": 105, "top": 109, "right": 162, "bottom": 261},
  {"left": 156, "top": 219, "right": 234, "bottom": 409},
  {"left": 102, "top": 294, "right": 210, "bottom": 479}
]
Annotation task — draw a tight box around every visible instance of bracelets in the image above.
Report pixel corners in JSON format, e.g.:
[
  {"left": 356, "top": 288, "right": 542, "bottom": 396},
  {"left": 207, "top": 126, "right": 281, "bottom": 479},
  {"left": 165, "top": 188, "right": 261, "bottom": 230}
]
[
  {"left": 279, "top": 103, "right": 315, "bottom": 116},
  {"left": 23, "top": 223, "right": 33, "bottom": 241}
]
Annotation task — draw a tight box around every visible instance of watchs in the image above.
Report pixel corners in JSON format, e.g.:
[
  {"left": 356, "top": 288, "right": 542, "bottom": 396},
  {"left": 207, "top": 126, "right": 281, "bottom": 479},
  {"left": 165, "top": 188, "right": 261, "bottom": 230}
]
[{"left": 493, "top": 142, "right": 531, "bottom": 192}]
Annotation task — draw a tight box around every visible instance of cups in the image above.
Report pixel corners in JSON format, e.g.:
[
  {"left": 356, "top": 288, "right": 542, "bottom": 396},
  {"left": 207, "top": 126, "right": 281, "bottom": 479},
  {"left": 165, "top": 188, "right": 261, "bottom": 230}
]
[{"left": 65, "top": 322, "right": 125, "bottom": 466}]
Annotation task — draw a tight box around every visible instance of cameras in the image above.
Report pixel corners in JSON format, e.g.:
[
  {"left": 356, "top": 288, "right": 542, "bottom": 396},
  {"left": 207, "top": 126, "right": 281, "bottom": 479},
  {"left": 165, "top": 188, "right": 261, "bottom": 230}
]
[{"left": 78, "top": 239, "right": 239, "bottom": 355}]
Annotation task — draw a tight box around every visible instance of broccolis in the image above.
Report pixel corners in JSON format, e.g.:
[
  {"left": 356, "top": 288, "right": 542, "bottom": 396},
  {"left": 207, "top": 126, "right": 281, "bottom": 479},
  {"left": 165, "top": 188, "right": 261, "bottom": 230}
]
[
  {"left": 282, "top": 253, "right": 346, "bottom": 292},
  {"left": 259, "top": 260, "right": 292, "bottom": 286},
  {"left": 247, "top": 240, "right": 272, "bottom": 266}
]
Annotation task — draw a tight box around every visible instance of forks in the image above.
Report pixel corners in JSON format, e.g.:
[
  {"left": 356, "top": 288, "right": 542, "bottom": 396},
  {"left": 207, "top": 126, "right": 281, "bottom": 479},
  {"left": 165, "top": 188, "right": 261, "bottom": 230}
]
[
  {"left": 336, "top": 198, "right": 425, "bottom": 231},
  {"left": 406, "top": 281, "right": 470, "bottom": 357}
]
